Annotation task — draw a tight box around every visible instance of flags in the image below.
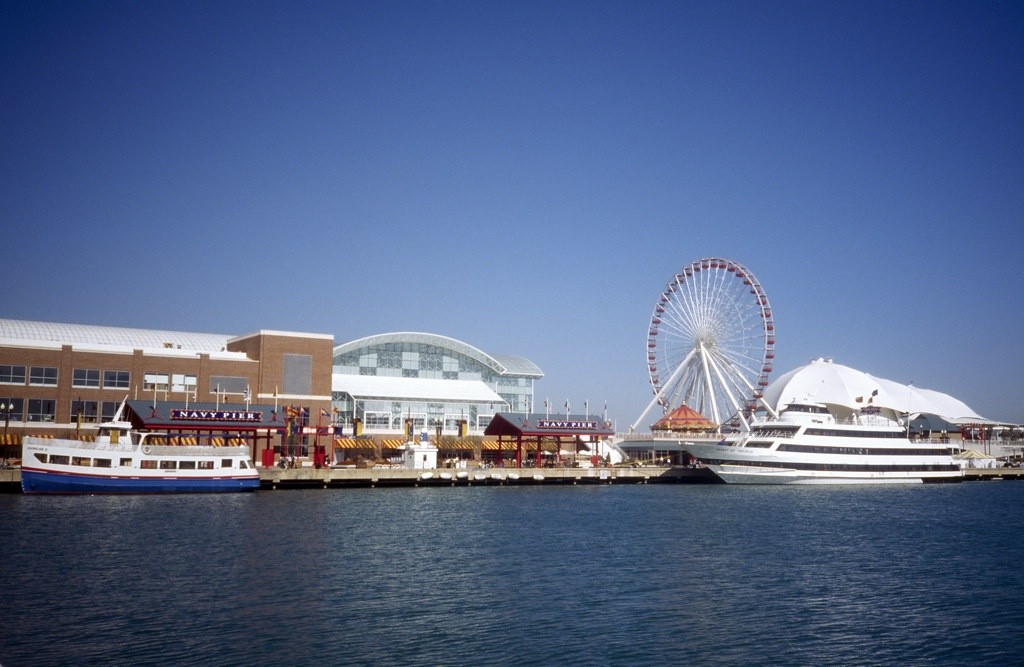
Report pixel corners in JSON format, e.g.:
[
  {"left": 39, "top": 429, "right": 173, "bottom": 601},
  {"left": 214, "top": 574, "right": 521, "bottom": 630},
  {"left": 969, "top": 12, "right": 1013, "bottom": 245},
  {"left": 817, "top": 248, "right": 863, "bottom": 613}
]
[
  {"left": 856, "top": 396, "right": 862, "bottom": 403},
  {"left": 868, "top": 389, "right": 878, "bottom": 405}
]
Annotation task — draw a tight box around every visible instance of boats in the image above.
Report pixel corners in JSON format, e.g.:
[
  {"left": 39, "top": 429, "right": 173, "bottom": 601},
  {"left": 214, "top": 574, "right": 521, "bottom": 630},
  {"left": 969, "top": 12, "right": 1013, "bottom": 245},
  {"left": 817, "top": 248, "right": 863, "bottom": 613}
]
[
  {"left": 20, "top": 436, "right": 261, "bottom": 492},
  {"left": 679, "top": 401, "right": 967, "bottom": 486}
]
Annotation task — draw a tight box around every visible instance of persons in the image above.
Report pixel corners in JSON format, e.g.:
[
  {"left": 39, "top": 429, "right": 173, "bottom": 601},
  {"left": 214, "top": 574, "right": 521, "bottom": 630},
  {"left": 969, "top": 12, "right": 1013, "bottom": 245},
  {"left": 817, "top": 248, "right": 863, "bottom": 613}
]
[
  {"left": 446, "top": 448, "right": 702, "bottom": 470},
  {"left": 277, "top": 452, "right": 295, "bottom": 470},
  {"left": 316, "top": 454, "right": 329, "bottom": 469}
]
